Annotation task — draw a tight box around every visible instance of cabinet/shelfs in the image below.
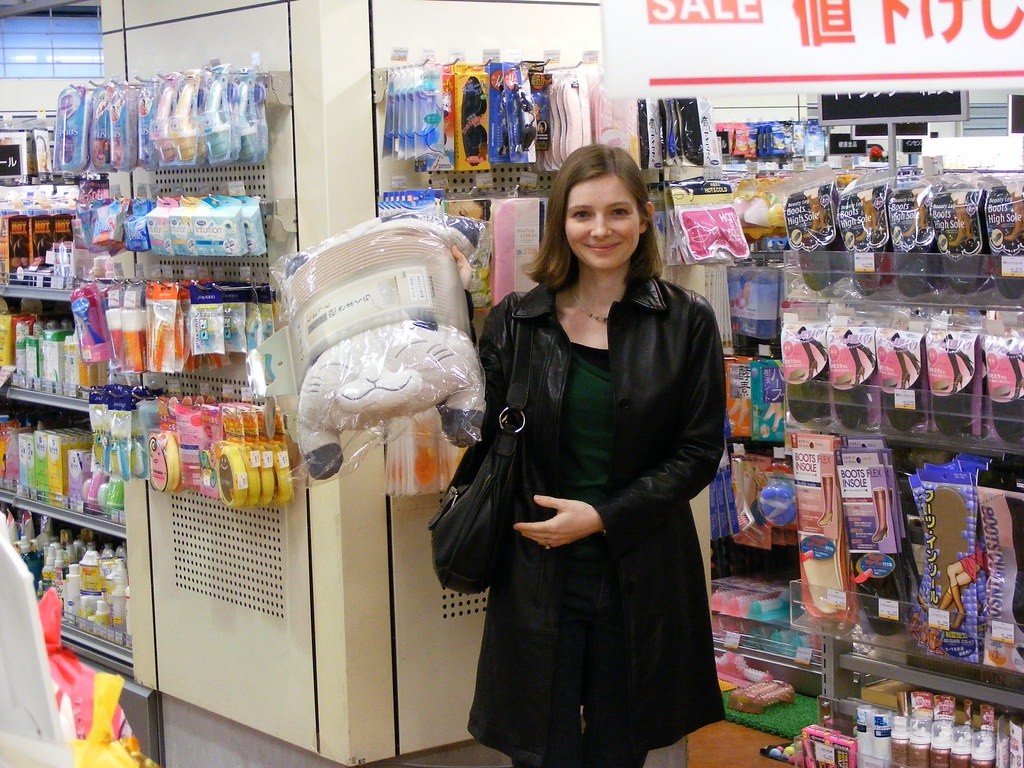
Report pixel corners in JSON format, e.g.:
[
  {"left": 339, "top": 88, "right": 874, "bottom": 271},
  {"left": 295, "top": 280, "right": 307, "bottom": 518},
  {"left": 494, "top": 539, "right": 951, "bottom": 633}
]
[
  {"left": 788, "top": 251, "right": 1024, "bottom": 757},
  {"left": 0, "top": 284, "right": 166, "bottom": 768}
]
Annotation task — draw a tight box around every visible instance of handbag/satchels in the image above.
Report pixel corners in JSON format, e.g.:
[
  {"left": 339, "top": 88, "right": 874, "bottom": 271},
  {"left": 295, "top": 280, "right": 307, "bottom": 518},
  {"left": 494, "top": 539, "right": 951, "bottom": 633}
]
[{"left": 427, "top": 406, "right": 527, "bottom": 594}]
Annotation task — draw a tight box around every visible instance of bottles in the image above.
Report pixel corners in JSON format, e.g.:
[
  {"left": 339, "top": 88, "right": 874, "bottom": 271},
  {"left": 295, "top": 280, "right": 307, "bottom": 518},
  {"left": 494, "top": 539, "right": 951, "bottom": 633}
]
[
  {"left": 6, "top": 511, "right": 132, "bottom": 644},
  {"left": 853, "top": 709, "right": 996, "bottom": 768}
]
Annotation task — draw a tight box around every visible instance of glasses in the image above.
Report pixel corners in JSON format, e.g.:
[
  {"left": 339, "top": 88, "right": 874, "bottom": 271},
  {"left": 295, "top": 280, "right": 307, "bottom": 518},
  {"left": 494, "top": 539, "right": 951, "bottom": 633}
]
[{"left": 388, "top": 68, "right": 553, "bottom": 176}]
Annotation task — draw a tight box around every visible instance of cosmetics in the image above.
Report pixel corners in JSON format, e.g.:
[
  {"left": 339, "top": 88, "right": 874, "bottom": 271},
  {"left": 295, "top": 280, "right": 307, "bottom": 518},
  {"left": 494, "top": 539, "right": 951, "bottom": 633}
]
[
  {"left": 1, "top": 215, "right": 144, "bottom": 647},
  {"left": 857, "top": 705, "right": 1023, "bottom": 768}
]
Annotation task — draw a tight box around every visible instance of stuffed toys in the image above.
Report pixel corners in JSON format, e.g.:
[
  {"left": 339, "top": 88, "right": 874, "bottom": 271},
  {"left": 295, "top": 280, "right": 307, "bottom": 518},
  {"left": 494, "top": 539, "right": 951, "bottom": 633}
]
[{"left": 282, "top": 214, "right": 486, "bottom": 481}]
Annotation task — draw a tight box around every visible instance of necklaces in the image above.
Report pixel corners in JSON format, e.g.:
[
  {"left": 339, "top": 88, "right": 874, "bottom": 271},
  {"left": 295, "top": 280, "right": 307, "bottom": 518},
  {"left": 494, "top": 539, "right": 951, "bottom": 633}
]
[{"left": 568, "top": 285, "right": 607, "bottom": 323}]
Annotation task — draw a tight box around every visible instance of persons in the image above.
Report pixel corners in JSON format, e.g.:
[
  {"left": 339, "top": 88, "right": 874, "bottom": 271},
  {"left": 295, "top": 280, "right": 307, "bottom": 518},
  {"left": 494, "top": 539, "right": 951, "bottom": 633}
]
[
  {"left": 27, "top": 136, "right": 50, "bottom": 174},
  {"left": 427, "top": 142, "right": 730, "bottom": 768}
]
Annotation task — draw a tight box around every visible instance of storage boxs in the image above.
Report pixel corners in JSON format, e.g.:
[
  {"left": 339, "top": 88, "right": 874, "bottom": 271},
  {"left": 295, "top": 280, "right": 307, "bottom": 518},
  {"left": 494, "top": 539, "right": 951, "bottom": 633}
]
[
  {"left": 1, "top": 126, "right": 83, "bottom": 283},
  {"left": 16, "top": 319, "right": 108, "bottom": 510},
  {"left": 715, "top": 264, "right": 786, "bottom": 447}
]
